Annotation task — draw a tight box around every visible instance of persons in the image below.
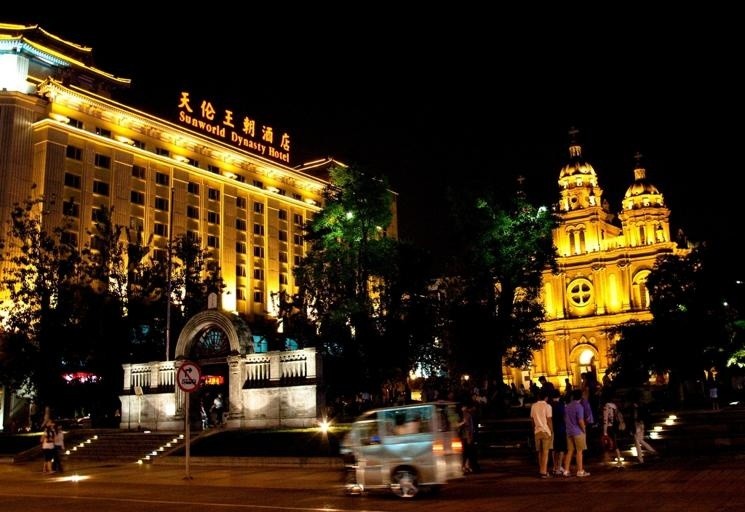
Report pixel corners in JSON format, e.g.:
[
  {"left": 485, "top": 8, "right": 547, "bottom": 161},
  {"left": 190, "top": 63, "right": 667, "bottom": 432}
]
[
  {"left": 333, "top": 373, "right": 674, "bottom": 482},
  {"left": 51, "top": 425, "right": 65, "bottom": 473},
  {"left": 709, "top": 385, "right": 721, "bottom": 410},
  {"left": 200, "top": 391, "right": 214, "bottom": 430},
  {"left": 212, "top": 393, "right": 224, "bottom": 425},
  {"left": 39, "top": 423, "right": 56, "bottom": 473}
]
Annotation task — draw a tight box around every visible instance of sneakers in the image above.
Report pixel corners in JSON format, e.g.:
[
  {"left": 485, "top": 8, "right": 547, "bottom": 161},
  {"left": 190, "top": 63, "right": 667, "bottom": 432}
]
[
  {"left": 540, "top": 471, "right": 553, "bottom": 479},
  {"left": 577, "top": 470, "right": 590, "bottom": 477},
  {"left": 553, "top": 466, "right": 570, "bottom": 476}
]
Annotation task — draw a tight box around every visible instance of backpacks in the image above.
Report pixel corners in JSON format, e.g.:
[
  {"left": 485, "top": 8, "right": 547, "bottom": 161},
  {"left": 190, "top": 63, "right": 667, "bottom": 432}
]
[{"left": 615, "top": 411, "right": 626, "bottom": 431}]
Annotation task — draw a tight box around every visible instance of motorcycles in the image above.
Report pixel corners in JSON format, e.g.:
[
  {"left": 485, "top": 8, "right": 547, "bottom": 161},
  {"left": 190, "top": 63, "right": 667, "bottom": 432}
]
[{"left": 339, "top": 400, "right": 466, "bottom": 499}]
